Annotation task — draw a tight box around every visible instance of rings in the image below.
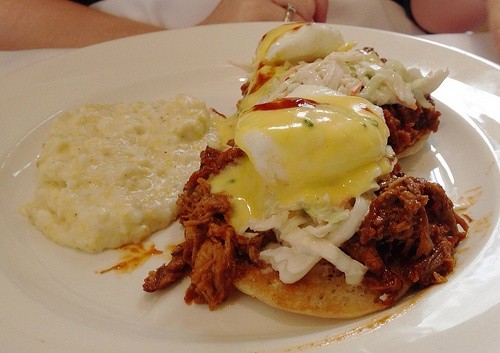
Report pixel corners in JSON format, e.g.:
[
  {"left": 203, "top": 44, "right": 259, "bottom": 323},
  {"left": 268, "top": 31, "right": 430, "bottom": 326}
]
[{"left": 280, "top": 3, "right": 296, "bottom": 22}]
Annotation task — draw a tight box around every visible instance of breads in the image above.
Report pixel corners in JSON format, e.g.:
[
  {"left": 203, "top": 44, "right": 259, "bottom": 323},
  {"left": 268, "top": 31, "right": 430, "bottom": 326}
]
[{"left": 233, "top": 256, "right": 414, "bottom": 319}]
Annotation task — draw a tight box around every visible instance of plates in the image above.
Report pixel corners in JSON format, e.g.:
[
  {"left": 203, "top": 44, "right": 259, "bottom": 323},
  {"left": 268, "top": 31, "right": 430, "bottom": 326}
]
[{"left": 0, "top": 21, "right": 500, "bottom": 352}]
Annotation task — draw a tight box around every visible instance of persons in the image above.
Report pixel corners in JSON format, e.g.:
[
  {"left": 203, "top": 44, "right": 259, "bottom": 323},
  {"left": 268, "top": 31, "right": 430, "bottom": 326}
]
[{"left": 0, "top": 0, "right": 500, "bottom": 48}]
